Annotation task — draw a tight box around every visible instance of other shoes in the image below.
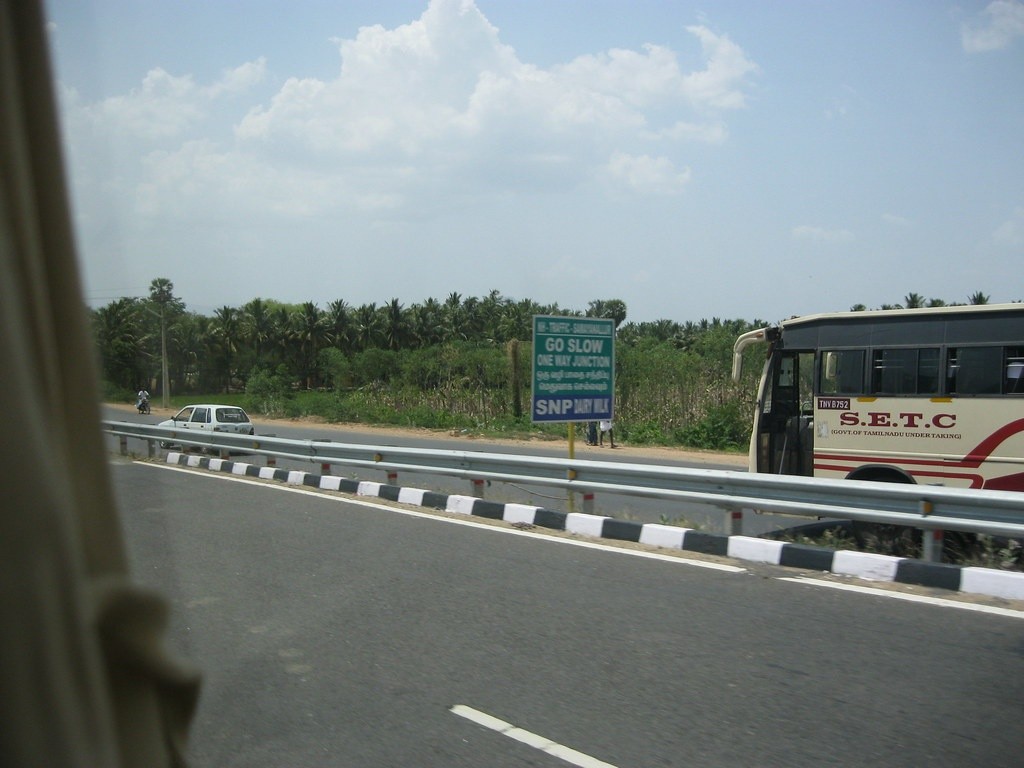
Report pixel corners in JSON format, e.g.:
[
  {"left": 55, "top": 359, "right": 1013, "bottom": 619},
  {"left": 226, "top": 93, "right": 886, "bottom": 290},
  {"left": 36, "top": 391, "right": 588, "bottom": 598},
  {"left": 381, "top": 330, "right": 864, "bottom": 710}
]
[
  {"left": 611, "top": 445, "right": 618, "bottom": 448},
  {"left": 600, "top": 445, "right": 604, "bottom": 448}
]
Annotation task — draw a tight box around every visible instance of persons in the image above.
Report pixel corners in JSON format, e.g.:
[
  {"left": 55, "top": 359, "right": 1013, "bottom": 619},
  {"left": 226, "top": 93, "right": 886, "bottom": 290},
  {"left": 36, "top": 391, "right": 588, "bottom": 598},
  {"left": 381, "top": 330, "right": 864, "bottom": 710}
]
[
  {"left": 599, "top": 419, "right": 619, "bottom": 448},
  {"left": 585, "top": 421, "right": 599, "bottom": 446},
  {"left": 137, "top": 387, "right": 152, "bottom": 414}
]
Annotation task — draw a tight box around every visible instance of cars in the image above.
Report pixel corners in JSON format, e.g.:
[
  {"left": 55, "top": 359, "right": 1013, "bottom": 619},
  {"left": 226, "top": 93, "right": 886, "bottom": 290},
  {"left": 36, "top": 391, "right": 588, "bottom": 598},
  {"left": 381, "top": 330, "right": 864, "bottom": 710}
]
[{"left": 157, "top": 403, "right": 254, "bottom": 455}]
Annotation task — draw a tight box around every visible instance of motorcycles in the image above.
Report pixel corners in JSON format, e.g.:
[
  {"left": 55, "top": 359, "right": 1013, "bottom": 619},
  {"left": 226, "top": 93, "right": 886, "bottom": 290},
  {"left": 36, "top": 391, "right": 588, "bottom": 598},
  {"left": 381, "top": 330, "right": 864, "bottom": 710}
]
[{"left": 137, "top": 397, "right": 151, "bottom": 415}]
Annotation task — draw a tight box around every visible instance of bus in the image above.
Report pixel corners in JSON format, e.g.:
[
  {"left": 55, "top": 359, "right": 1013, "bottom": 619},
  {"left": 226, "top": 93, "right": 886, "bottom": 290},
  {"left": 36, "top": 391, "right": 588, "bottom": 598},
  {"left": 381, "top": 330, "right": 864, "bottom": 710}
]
[{"left": 732, "top": 301, "right": 1024, "bottom": 497}]
